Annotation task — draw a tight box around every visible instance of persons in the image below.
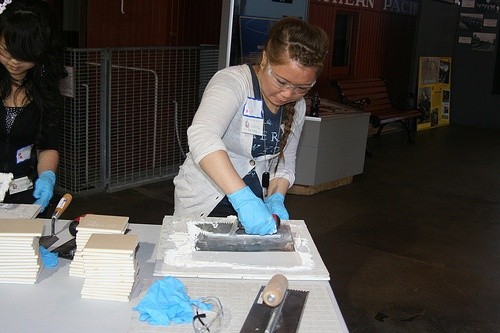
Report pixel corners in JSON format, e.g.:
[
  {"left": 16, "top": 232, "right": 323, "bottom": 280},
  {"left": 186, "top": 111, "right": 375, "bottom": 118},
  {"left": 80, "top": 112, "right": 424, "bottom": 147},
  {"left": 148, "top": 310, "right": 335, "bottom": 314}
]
[
  {"left": 0, "top": 1, "right": 65, "bottom": 221},
  {"left": 173, "top": 18, "right": 329, "bottom": 235}
]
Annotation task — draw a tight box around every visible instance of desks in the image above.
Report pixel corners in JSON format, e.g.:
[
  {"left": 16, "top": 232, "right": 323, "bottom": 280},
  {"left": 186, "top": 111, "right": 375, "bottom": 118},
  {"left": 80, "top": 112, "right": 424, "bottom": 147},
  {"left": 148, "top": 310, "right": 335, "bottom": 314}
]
[{"left": 0, "top": 219, "right": 351, "bottom": 333}]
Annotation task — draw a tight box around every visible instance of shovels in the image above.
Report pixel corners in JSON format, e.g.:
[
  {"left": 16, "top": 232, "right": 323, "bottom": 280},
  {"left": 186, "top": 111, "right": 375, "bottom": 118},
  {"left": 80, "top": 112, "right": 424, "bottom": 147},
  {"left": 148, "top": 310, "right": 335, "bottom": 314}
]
[
  {"left": 39, "top": 193, "right": 73, "bottom": 250},
  {"left": 50, "top": 214, "right": 86, "bottom": 260}
]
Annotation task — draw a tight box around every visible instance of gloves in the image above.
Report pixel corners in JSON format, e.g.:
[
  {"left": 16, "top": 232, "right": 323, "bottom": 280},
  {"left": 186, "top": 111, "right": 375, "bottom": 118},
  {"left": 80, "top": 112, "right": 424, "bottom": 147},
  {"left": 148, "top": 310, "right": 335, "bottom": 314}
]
[
  {"left": 226, "top": 185, "right": 277, "bottom": 235},
  {"left": 265, "top": 192, "right": 289, "bottom": 220},
  {"left": 131, "top": 275, "right": 212, "bottom": 325},
  {"left": 33, "top": 170, "right": 56, "bottom": 213}
]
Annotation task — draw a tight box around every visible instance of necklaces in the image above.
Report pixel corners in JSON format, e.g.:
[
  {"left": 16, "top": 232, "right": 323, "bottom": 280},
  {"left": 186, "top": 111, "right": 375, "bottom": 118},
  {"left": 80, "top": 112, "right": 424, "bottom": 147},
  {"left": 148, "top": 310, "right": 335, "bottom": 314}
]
[
  {"left": 258, "top": 72, "right": 284, "bottom": 187},
  {"left": 4, "top": 100, "right": 17, "bottom": 120}
]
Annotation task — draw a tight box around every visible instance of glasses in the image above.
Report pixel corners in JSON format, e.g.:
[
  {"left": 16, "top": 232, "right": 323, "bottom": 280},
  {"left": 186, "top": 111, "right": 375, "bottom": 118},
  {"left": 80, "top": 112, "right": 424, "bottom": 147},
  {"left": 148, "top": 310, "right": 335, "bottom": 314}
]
[{"left": 267, "top": 55, "right": 316, "bottom": 93}]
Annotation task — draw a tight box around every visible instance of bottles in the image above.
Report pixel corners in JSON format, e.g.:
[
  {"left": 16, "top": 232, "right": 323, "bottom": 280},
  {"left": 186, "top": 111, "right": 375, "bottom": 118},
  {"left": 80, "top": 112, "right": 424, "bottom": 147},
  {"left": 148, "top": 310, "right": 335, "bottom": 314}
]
[{"left": 310, "top": 93, "right": 321, "bottom": 118}]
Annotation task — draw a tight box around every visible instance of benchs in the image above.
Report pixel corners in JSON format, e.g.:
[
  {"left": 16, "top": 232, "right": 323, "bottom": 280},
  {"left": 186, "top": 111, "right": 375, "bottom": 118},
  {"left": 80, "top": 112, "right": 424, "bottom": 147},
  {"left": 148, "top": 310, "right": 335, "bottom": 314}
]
[{"left": 333, "top": 78, "right": 423, "bottom": 145}]
